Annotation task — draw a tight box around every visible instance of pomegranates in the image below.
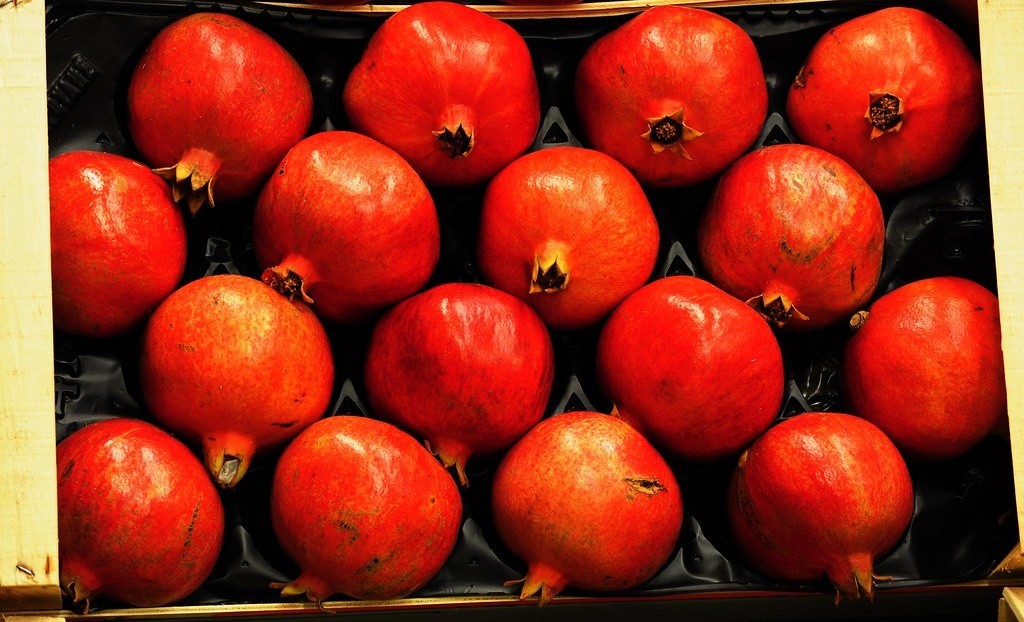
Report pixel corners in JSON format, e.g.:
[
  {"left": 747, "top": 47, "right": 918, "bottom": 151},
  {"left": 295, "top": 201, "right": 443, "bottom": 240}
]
[{"left": 49, "top": 6, "right": 1006, "bottom": 611}]
